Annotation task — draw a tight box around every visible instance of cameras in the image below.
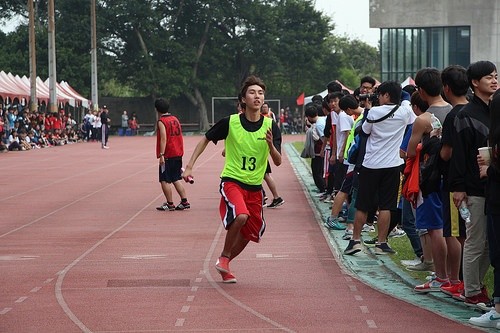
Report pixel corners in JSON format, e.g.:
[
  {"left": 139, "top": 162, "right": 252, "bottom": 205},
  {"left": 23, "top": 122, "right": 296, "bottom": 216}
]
[{"left": 359, "top": 93, "right": 380, "bottom": 105}]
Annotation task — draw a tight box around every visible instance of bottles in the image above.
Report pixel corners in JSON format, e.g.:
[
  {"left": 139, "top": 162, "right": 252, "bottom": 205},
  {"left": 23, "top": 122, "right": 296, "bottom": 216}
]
[
  {"left": 459, "top": 200, "right": 471, "bottom": 224},
  {"left": 430, "top": 114, "right": 443, "bottom": 140}
]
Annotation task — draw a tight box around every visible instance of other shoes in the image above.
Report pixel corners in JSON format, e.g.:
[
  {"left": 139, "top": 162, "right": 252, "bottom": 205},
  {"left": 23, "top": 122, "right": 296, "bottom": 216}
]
[
  {"left": 400, "top": 258, "right": 421, "bottom": 266},
  {"left": 316, "top": 189, "right": 348, "bottom": 222}
]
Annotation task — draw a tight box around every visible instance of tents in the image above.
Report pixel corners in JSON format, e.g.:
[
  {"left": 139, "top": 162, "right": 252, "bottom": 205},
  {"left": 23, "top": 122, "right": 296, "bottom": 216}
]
[
  {"left": 0, "top": 70, "right": 92, "bottom": 110},
  {"left": 401, "top": 76, "right": 417, "bottom": 89},
  {"left": 302, "top": 79, "right": 355, "bottom": 134},
  {"left": 373, "top": 78, "right": 382, "bottom": 87}
]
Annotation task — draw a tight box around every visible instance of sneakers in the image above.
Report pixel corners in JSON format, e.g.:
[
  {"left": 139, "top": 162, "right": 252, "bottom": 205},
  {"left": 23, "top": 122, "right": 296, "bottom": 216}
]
[
  {"left": 414, "top": 274, "right": 450, "bottom": 292},
  {"left": 325, "top": 216, "right": 347, "bottom": 230},
  {"left": 344, "top": 239, "right": 361, "bottom": 255},
  {"left": 363, "top": 235, "right": 389, "bottom": 246},
  {"left": 214, "top": 257, "right": 230, "bottom": 273},
  {"left": 452, "top": 293, "right": 488, "bottom": 305},
  {"left": 464, "top": 292, "right": 490, "bottom": 306},
  {"left": 156, "top": 203, "right": 175, "bottom": 211},
  {"left": 342, "top": 228, "right": 353, "bottom": 239},
  {"left": 362, "top": 224, "right": 375, "bottom": 232},
  {"left": 440, "top": 281, "right": 464, "bottom": 295},
  {"left": 263, "top": 196, "right": 268, "bottom": 207},
  {"left": 477, "top": 298, "right": 495, "bottom": 312},
  {"left": 390, "top": 227, "right": 406, "bottom": 237},
  {"left": 220, "top": 271, "right": 237, "bottom": 283},
  {"left": 469, "top": 309, "right": 500, "bottom": 327},
  {"left": 374, "top": 242, "right": 396, "bottom": 254},
  {"left": 175, "top": 201, "right": 190, "bottom": 210},
  {"left": 405, "top": 262, "right": 435, "bottom": 273},
  {"left": 266, "top": 197, "right": 284, "bottom": 208}
]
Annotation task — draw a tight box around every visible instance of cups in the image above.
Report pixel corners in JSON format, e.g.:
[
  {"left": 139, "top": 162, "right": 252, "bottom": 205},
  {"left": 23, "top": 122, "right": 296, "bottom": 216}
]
[{"left": 478, "top": 146, "right": 493, "bottom": 165}]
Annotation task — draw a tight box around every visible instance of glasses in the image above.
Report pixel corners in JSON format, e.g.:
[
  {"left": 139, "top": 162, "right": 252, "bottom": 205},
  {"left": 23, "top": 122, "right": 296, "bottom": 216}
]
[{"left": 414, "top": 86, "right": 419, "bottom": 90}]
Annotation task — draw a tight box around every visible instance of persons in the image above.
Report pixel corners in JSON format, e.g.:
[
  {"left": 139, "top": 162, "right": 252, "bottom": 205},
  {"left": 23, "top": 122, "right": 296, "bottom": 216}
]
[
  {"left": 121, "top": 110, "right": 138, "bottom": 136},
  {"left": 180, "top": 61, "right": 500, "bottom": 330},
  {"left": 0, "top": 102, "right": 111, "bottom": 152},
  {"left": 154, "top": 98, "right": 190, "bottom": 211}
]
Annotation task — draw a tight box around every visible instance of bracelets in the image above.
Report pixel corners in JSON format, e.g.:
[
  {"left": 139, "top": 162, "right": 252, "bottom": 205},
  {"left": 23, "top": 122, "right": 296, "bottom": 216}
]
[{"left": 160, "top": 153, "right": 164, "bottom": 156}]
